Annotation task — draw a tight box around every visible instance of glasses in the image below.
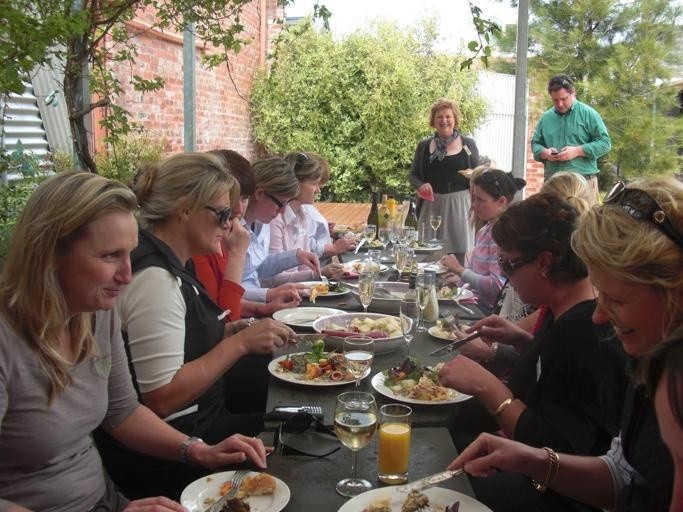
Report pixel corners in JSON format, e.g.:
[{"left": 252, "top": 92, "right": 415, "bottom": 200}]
[
  {"left": 203, "top": 205, "right": 232, "bottom": 227},
  {"left": 548, "top": 78, "right": 571, "bottom": 89},
  {"left": 263, "top": 190, "right": 294, "bottom": 208},
  {"left": 601, "top": 180, "right": 683, "bottom": 249},
  {"left": 273, "top": 410, "right": 341, "bottom": 459},
  {"left": 497, "top": 251, "right": 539, "bottom": 276},
  {"left": 480, "top": 169, "right": 506, "bottom": 196},
  {"left": 294, "top": 152, "right": 309, "bottom": 174}
]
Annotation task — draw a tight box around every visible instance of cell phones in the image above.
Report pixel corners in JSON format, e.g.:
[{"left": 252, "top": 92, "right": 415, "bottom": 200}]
[{"left": 274, "top": 404, "right": 325, "bottom": 420}]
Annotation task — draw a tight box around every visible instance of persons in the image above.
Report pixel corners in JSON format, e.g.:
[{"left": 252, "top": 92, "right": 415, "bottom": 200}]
[
  {"left": 455, "top": 164, "right": 493, "bottom": 247},
  {"left": 298, "top": 152, "right": 358, "bottom": 261},
  {"left": 529, "top": 71, "right": 611, "bottom": 204},
  {"left": 446, "top": 168, "right": 681, "bottom": 510},
  {"left": 438, "top": 167, "right": 526, "bottom": 314},
  {"left": 258, "top": 151, "right": 347, "bottom": 289},
  {"left": 115, "top": 150, "right": 298, "bottom": 448},
  {"left": 184, "top": 149, "right": 308, "bottom": 415},
  {"left": 436, "top": 189, "right": 638, "bottom": 451},
  {"left": 240, "top": 154, "right": 323, "bottom": 302},
  {"left": 409, "top": 97, "right": 486, "bottom": 269},
  {"left": 500, "top": 164, "right": 597, "bottom": 320},
  {"left": 642, "top": 336, "right": 682, "bottom": 511},
  {"left": 0, "top": 170, "right": 276, "bottom": 512}
]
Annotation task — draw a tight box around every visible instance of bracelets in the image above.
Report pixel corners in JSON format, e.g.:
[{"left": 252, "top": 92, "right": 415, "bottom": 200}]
[
  {"left": 487, "top": 395, "right": 517, "bottom": 422},
  {"left": 242, "top": 316, "right": 255, "bottom": 329},
  {"left": 177, "top": 433, "right": 207, "bottom": 466},
  {"left": 530, "top": 443, "right": 561, "bottom": 497},
  {"left": 229, "top": 317, "right": 240, "bottom": 335},
  {"left": 253, "top": 302, "right": 263, "bottom": 314}
]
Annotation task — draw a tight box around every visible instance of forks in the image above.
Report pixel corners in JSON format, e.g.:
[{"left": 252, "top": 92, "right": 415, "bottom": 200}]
[
  {"left": 441, "top": 308, "right": 462, "bottom": 329},
  {"left": 203, "top": 464, "right": 250, "bottom": 510}
]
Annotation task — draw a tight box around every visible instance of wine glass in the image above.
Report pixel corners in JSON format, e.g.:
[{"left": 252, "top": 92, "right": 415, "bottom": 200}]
[
  {"left": 352, "top": 216, "right": 444, "bottom": 360},
  {"left": 333, "top": 392, "right": 378, "bottom": 497},
  {"left": 343, "top": 335, "right": 372, "bottom": 388}
]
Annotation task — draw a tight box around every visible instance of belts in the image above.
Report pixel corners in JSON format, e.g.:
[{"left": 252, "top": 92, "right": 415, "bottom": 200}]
[{"left": 584, "top": 174, "right": 597, "bottom": 180}]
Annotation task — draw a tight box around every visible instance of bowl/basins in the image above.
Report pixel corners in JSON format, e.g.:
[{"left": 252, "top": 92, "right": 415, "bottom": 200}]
[{"left": 312, "top": 311, "right": 413, "bottom": 356}]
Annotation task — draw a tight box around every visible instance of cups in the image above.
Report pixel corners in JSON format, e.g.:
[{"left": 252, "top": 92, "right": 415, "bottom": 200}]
[{"left": 374, "top": 403, "right": 412, "bottom": 486}]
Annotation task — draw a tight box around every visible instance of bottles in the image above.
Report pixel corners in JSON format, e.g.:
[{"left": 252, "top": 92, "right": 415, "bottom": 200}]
[{"left": 364, "top": 190, "right": 421, "bottom": 231}]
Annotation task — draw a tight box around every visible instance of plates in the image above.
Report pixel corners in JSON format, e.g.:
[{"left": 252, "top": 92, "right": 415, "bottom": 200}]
[
  {"left": 438, "top": 286, "right": 476, "bottom": 303},
  {"left": 426, "top": 325, "right": 472, "bottom": 343},
  {"left": 179, "top": 470, "right": 292, "bottom": 511},
  {"left": 338, "top": 260, "right": 388, "bottom": 276},
  {"left": 273, "top": 305, "right": 345, "bottom": 330},
  {"left": 372, "top": 366, "right": 479, "bottom": 404},
  {"left": 339, "top": 482, "right": 493, "bottom": 512},
  {"left": 295, "top": 282, "right": 350, "bottom": 299},
  {"left": 266, "top": 351, "right": 369, "bottom": 389}
]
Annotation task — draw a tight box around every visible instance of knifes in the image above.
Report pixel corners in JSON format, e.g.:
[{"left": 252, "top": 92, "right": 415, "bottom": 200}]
[
  {"left": 430, "top": 333, "right": 485, "bottom": 359},
  {"left": 410, "top": 462, "right": 468, "bottom": 489}
]
